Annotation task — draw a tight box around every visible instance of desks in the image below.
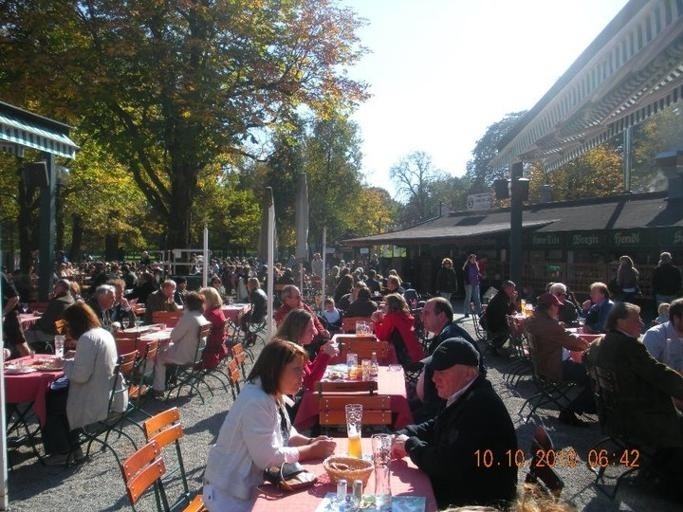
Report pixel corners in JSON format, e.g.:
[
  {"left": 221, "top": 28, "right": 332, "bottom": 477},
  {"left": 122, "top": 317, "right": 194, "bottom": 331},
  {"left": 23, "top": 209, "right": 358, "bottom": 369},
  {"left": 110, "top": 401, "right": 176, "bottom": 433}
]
[{"left": 2, "top": 302, "right": 437, "bottom": 512}]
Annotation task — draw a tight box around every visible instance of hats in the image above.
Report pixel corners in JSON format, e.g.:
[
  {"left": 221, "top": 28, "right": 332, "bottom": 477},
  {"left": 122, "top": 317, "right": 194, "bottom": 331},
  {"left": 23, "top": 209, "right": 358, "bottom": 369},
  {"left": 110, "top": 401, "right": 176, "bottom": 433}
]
[
  {"left": 418, "top": 336, "right": 479, "bottom": 371},
  {"left": 537, "top": 293, "right": 566, "bottom": 307}
]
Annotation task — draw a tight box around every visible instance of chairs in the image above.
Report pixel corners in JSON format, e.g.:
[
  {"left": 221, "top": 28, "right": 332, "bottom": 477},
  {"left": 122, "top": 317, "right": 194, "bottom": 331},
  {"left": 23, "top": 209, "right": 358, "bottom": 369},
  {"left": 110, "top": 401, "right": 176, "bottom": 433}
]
[{"left": 469, "top": 301, "right": 682, "bottom": 503}]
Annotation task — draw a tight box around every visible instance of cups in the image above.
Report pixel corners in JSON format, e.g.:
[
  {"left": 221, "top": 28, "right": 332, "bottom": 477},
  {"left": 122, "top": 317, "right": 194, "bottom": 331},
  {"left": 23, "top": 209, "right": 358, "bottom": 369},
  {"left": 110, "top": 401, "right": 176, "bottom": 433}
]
[
  {"left": 577, "top": 326, "right": 585, "bottom": 335},
  {"left": 55, "top": 334, "right": 67, "bottom": 360},
  {"left": 355, "top": 320, "right": 373, "bottom": 337},
  {"left": 344, "top": 403, "right": 363, "bottom": 462},
  {"left": 509, "top": 298, "right": 536, "bottom": 320},
  {"left": 375, "top": 465, "right": 393, "bottom": 512},
  {"left": 371, "top": 434, "right": 392, "bottom": 465}
]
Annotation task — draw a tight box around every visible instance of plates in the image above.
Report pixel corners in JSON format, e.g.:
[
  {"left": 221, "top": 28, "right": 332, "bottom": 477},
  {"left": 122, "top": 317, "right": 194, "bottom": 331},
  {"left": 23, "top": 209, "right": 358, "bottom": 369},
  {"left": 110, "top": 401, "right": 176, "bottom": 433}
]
[{"left": 5, "top": 361, "right": 66, "bottom": 374}]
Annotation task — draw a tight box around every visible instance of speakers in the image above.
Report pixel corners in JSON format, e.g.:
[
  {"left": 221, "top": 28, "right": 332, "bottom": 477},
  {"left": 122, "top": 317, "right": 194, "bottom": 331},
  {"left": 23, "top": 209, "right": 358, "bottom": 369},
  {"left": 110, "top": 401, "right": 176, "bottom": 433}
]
[
  {"left": 32, "top": 160, "right": 49, "bottom": 187},
  {"left": 495, "top": 179, "right": 508, "bottom": 199},
  {"left": 517, "top": 177, "right": 530, "bottom": 200}
]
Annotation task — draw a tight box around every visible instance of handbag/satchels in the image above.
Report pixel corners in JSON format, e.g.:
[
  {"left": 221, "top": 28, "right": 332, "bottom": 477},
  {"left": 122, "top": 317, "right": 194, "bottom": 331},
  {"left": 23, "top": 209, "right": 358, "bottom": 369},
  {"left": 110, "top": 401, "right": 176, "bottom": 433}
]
[{"left": 263, "top": 461, "right": 319, "bottom": 492}]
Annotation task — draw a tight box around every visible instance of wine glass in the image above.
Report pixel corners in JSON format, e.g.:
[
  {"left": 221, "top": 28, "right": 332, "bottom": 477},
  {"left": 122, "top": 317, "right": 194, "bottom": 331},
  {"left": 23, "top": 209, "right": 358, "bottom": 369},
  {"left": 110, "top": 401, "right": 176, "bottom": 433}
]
[
  {"left": 222, "top": 304, "right": 248, "bottom": 309},
  {"left": 123, "top": 316, "right": 130, "bottom": 332},
  {"left": 133, "top": 316, "right": 141, "bottom": 331},
  {"left": 22, "top": 303, "right": 29, "bottom": 318}
]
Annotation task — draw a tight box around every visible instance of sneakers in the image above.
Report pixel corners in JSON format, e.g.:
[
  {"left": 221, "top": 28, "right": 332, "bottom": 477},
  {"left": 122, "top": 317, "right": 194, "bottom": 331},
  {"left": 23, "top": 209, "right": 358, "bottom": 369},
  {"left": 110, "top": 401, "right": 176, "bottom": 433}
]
[
  {"left": 145, "top": 389, "right": 164, "bottom": 397},
  {"left": 557, "top": 410, "right": 589, "bottom": 427},
  {"left": 42, "top": 448, "right": 86, "bottom": 466}
]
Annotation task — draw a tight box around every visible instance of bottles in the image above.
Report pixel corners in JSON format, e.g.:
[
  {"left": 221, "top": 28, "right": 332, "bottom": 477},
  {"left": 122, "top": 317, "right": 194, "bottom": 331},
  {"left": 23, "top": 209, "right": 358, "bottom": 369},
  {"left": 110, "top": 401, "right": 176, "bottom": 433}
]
[
  {"left": 331, "top": 478, "right": 362, "bottom": 512},
  {"left": 329, "top": 352, "right": 379, "bottom": 381}
]
